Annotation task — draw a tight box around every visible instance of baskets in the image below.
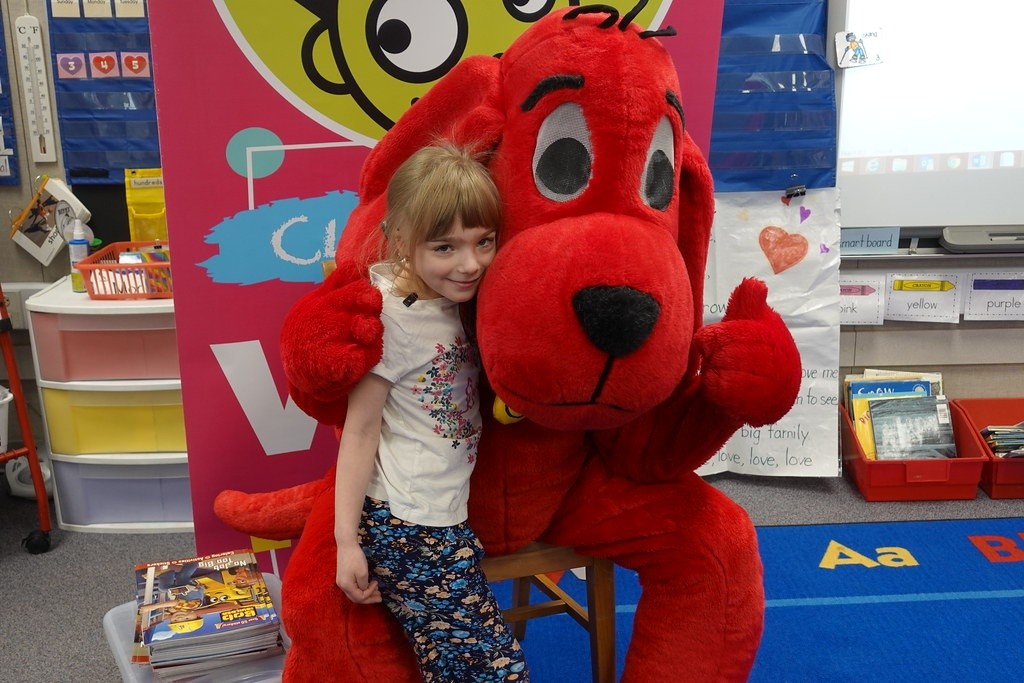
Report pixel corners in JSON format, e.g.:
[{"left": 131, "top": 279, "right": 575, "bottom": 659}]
[{"left": 76, "top": 241, "right": 173, "bottom": 300}]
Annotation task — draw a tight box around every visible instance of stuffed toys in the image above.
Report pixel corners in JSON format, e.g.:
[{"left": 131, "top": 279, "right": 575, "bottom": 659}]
[{"left": 211, "top": 0, "right": 803, "bottom": 683}]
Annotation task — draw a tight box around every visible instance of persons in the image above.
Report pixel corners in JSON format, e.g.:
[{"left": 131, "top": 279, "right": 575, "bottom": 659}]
[{"left": 334, "top": 144, "right": 520, "bottom": 642}]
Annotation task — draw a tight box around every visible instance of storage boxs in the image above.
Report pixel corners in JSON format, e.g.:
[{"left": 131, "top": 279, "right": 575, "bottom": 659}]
[
  {"left": 840, "top": 397, "right": 989, "bottom": 501},
  {"left": 954, "top": 397, "right": 1024, "bottom": 500},
  {"left": 0, "top": 385, "right": 14, "bottom": 453},
  {"left": 75, "top": 240, "right": 172, "bottom": 300}
]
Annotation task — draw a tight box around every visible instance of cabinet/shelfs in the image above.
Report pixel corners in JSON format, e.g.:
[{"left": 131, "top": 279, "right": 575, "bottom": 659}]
[{"left": 24, "top": 273, "right": 195, "bottom": 535}]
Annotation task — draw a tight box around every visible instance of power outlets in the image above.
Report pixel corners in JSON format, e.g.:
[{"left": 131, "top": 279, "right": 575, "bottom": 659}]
[{"left": 0, "top": 289, "right": 24, "bottom": 331}]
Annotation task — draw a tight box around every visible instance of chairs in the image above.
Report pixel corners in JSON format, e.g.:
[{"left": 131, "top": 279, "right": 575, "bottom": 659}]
[{"left": 322, "top": 261, "right": 616, "bottom": 683}]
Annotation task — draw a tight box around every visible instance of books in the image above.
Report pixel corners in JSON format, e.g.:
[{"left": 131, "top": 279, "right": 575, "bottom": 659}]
[
  {"left": 847, "top": 366, "right": 1022, "bottom": 458},
  {"left": 129, "top": 547, "right": 285, "bottom": 683}
]
